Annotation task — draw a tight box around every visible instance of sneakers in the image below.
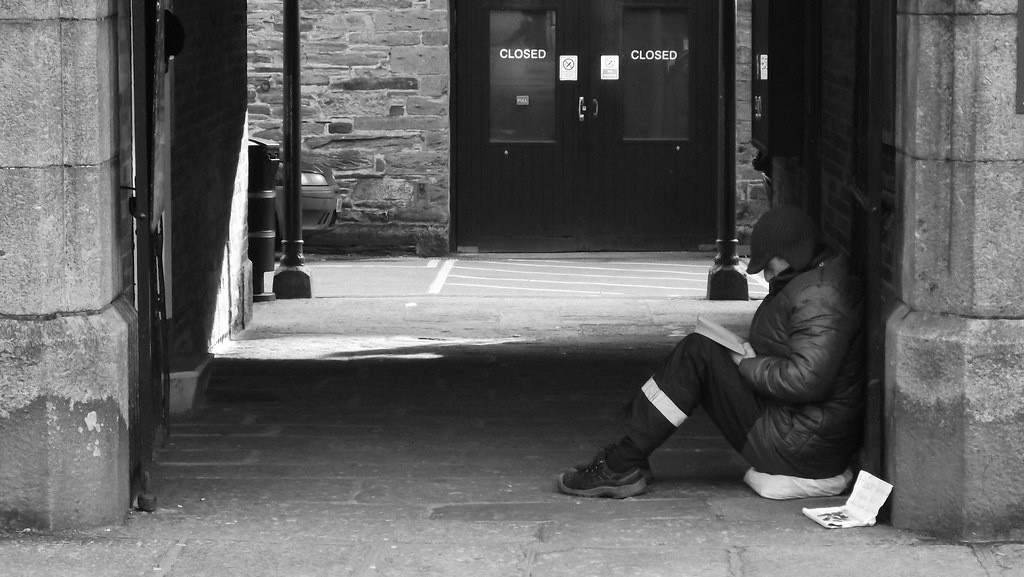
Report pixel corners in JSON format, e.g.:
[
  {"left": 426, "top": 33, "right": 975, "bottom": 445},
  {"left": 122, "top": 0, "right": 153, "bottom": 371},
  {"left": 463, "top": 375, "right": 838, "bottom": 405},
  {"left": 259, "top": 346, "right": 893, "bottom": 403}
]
[{"left": 559, "top": 451, "right": 656, "bottom": 499}]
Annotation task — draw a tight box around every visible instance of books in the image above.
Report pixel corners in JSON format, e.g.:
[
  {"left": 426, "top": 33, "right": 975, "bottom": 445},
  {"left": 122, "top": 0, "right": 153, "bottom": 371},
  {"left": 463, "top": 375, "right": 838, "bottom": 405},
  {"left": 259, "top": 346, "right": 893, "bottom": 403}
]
[{"left": 696, "top": 316, "right": 747, "bottom": 355}]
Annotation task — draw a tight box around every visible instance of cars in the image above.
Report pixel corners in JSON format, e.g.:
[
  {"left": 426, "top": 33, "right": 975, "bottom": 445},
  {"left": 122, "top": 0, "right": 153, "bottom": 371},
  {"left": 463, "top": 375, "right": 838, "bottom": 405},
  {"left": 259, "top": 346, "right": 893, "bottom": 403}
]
[{"left": 249, "top": 137, "right": 342, "bottom": 249}]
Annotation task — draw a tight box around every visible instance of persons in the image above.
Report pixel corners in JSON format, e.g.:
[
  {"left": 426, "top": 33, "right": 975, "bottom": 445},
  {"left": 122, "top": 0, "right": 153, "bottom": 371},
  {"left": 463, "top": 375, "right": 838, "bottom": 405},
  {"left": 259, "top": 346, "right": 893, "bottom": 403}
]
[{"left": 556, "top": 208, "right": 861, "bottom": 499}]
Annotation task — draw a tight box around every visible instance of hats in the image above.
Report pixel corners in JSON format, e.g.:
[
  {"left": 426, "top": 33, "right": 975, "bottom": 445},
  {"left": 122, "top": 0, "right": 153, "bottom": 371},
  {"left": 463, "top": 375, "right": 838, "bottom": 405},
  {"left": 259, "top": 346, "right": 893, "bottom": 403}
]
[{"left": 745, "top": 204, "right": 819, "bottom": 272}]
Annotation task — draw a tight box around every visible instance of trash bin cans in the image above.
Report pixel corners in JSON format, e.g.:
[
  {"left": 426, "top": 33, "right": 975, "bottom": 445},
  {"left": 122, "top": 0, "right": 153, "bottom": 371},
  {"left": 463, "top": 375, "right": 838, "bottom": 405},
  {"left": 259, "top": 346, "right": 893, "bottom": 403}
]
[{"left": 247, "top": 137, "right": 280, "bottom": 271}]
